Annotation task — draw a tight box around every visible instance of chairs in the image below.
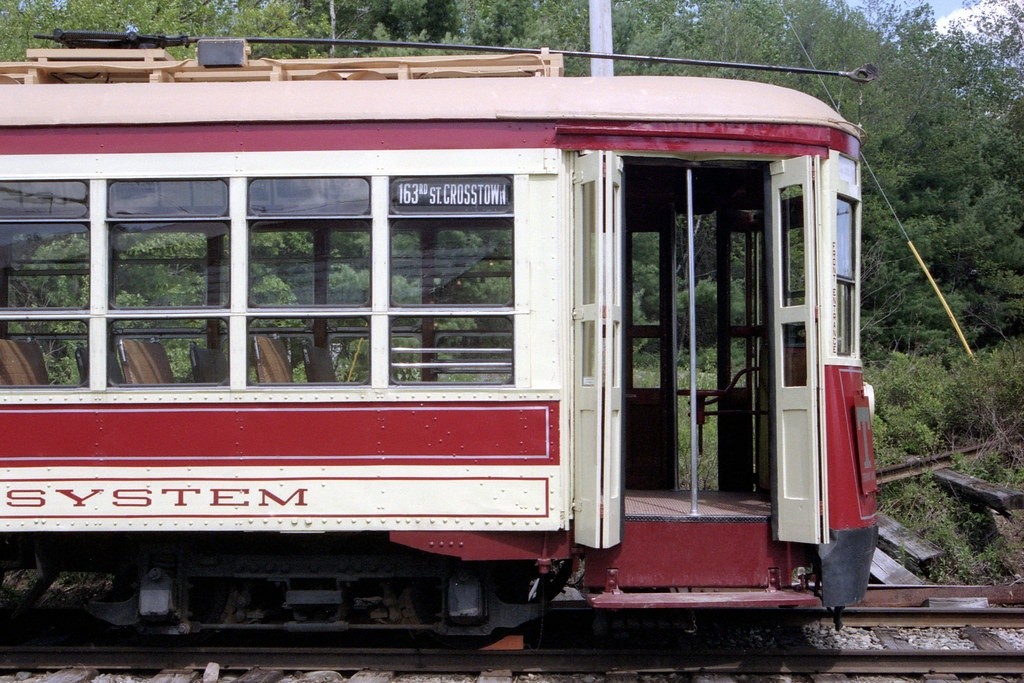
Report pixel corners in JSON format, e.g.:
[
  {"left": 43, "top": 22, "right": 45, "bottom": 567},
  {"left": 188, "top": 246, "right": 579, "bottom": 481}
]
[
  {"left": 0, "top": 338, "right": 49, "bottom": 385},
  {"left": 253, "top": 334, "right": 295, "bottom": 383},
  {"left": 119, "top": 338, "right": 174, "bottom": 384},
  {"left": 302, "top": 343, "right": 338, "bottom": 382},
  {"left": 77, "top": 347, "right": 124, "bottom": 385},
  {"left": 189, "top": 340, "right": 228, "bottom": 383}
]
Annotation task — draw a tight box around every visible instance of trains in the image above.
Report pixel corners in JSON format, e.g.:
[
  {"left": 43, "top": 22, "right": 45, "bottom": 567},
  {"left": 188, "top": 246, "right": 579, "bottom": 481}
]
[{"left": 0, "top": 27, "right": 879, "bottom": 636}]
